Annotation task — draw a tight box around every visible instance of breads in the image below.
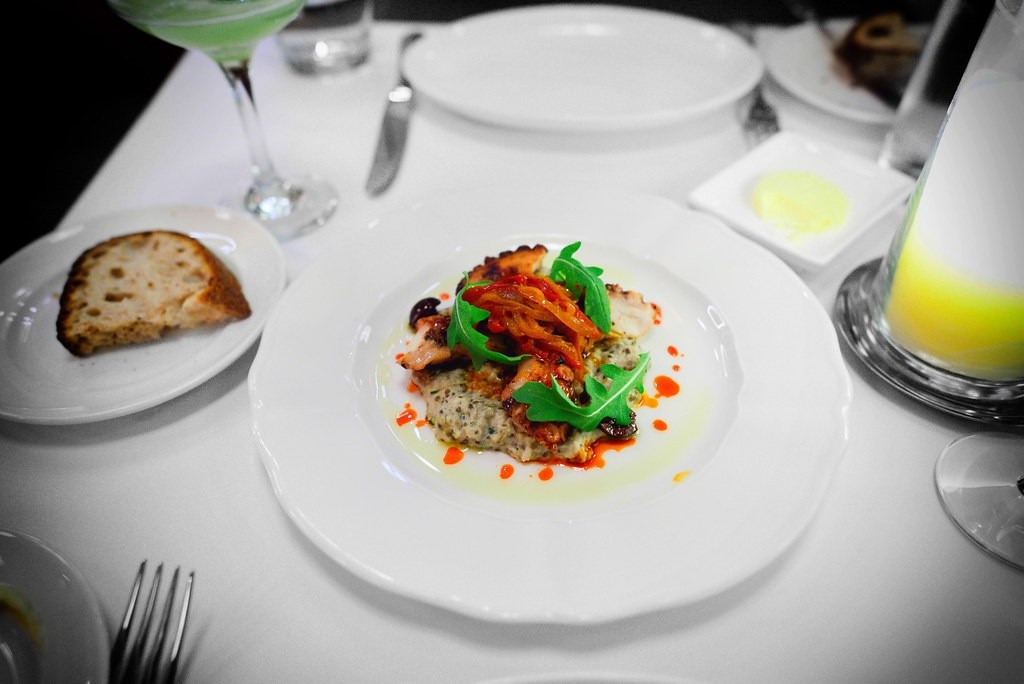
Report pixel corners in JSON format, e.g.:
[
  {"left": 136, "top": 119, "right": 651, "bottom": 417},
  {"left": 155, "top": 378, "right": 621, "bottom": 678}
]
[{"left": 56, "top": 229, "right": 250, "bottom": 357}]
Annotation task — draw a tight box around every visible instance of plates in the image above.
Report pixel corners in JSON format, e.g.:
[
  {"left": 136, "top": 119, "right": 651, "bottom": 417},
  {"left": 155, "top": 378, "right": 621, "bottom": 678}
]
[
  {"left": 689, "top": 133, "right": 915, "bottom": 261},
  {"left": 0, "top": 529, "right": 109, "bottom": 683},
  {"left": 0, "top": 198, "right": 288, "bottom": 427},
  {"left": 247, "top": 177, "right": 853, "bottom": 623},
  {"left": 762, "top": 17, "right": 892, "bottom": 126},
  {"left": 399, "top": 3, "right": 763, "bottom": 132}
]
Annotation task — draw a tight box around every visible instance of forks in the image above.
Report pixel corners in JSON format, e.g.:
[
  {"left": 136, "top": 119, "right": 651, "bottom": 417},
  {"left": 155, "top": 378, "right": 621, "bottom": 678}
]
[
  {"left": 110, "top": 559, "right": 194, "bottom": 684},
  {"left": 732, "top": 18, "right": 777, "bottom": 133}
]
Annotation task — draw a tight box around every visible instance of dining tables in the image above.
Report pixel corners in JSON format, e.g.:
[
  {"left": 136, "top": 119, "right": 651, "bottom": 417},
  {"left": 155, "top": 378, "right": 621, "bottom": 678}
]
[{"left": 0, "top": 21, "right": 1024, "bottom": 684}]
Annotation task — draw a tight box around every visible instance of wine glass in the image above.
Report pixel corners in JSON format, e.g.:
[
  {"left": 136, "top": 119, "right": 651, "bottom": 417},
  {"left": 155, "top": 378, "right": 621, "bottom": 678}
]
[{"left": 112, "top": 2, "right": 340, "bottom": 230}]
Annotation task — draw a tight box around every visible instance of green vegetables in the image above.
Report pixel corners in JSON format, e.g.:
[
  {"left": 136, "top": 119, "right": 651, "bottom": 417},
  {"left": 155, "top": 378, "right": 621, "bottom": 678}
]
[{"left": 445, "top": 240, "right": 652, "bottom": 433}]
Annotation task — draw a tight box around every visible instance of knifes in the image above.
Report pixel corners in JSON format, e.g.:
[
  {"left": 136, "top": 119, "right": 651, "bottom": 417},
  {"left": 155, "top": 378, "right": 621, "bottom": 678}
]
[{"left": 359, "top": 30, "right": 422, "bottom": 193}]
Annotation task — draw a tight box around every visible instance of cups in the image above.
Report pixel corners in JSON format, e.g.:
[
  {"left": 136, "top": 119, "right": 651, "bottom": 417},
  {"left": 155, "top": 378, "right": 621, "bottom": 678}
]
[{"left": 274, "top": 0, "right": 373, "bottom": 77}]
